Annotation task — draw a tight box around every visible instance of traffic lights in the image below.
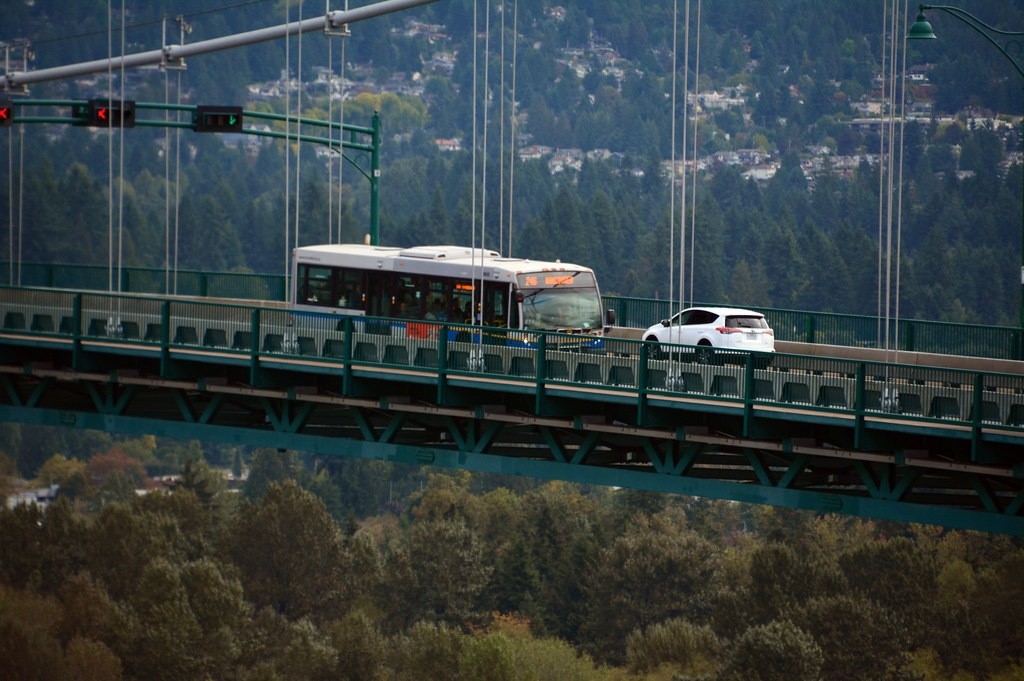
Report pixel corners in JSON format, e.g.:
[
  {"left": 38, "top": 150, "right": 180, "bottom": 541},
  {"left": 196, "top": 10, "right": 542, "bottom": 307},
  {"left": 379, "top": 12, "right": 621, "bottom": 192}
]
[
  {"left": 0, "top": 100, "right": 16, "bottom": 126},
  {"left": 87, "top": 97, "right": 136, "bottom": 129},
  {"left": 193, "top": 104, "right": 243, "bottom": 132}
]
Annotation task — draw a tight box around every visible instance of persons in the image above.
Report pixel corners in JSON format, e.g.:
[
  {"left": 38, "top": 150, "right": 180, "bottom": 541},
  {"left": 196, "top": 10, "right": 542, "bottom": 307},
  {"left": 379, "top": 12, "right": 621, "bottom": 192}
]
[
  {"left": 565, "top": 306, "right": 574, "bottom": 320},
  {"left": 308, "top": 275, "right": 471, "bottom": 323},
  {"left": 524, "top": 304, "right": 540, "bottom": 329}
]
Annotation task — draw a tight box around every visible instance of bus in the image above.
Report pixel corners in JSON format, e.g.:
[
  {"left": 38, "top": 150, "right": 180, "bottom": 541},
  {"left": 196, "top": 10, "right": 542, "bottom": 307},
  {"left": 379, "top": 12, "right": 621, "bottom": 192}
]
[{"left": 288, "top": 241, "right": 616, "bottom": 347}]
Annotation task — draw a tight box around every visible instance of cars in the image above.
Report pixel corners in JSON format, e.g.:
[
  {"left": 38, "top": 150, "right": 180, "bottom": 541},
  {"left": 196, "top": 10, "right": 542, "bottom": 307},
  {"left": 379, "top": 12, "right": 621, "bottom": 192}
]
[{"left": 641, "top": 306, "right": 776, "bottom": 367}]
